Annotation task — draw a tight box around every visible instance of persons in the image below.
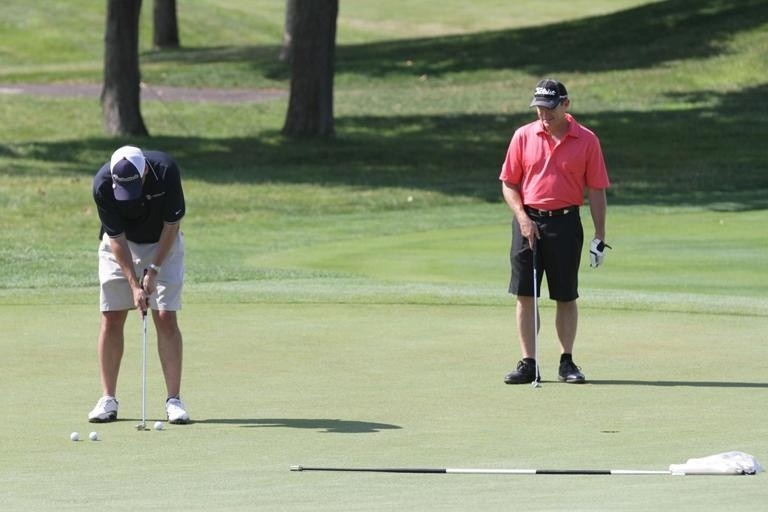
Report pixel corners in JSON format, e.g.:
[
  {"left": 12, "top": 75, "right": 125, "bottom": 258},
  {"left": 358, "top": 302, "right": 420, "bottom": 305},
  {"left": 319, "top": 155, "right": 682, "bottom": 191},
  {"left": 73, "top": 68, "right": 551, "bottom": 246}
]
[
  {"left": 498, "top": 79, "right": 611, "bottom": 386},
  {"left": 86, "top": 145, "right": 192, "bottom": 425}
]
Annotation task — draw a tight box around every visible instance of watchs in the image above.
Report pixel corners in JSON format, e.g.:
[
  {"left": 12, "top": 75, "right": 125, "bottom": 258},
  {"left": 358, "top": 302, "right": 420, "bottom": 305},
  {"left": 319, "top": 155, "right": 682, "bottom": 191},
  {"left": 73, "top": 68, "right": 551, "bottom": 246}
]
[{"left": 150, "top": 263, "right": 161, "bottom": 273}]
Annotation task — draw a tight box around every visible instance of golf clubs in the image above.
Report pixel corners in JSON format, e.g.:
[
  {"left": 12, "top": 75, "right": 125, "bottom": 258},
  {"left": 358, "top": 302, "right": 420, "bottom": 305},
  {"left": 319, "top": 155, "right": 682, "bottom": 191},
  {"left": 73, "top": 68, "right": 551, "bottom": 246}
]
[
  {"left": 532, "top": 234, "right": 540, "bottom": 388},
  {"left": 134, "top": 269, "right": 151, "bottom": 431}
]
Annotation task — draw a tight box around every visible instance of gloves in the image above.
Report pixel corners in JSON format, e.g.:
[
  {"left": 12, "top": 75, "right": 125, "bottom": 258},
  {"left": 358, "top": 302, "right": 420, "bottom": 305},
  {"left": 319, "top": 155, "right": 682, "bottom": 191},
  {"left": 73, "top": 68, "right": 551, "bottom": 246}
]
[{"left": 589, "top": 239, "right": 605, "bottom": 269}]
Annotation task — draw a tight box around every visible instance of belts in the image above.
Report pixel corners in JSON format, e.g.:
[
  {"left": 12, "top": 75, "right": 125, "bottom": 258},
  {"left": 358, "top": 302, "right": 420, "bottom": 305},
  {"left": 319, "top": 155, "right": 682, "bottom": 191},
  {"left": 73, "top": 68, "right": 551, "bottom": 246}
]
[{"left": 526, "top": 208, "right": 575, "bottom": 218}]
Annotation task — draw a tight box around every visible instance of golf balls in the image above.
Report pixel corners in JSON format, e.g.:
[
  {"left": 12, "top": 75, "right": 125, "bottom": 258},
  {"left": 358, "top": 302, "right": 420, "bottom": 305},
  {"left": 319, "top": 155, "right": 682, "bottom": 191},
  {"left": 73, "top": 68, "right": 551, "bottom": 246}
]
[
  {"left": 71, "top": 432, "right": 80, "bottom": 440},
  {"left": 89, "top": 432, "right": 98, "bottom": 440},
  {"left": 154, "top": 421, "right": 163, "bottom": 430}
]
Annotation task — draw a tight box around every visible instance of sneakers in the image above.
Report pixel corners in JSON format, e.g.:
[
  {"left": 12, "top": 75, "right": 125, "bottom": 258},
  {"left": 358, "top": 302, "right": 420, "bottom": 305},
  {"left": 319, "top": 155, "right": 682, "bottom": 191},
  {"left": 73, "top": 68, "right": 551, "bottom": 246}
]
[
  {"left": 88, "top": 397, "right": 118, "bottom": 422},
  {"left": 165, "top": 398, "right": 189, "bottom": 424}
]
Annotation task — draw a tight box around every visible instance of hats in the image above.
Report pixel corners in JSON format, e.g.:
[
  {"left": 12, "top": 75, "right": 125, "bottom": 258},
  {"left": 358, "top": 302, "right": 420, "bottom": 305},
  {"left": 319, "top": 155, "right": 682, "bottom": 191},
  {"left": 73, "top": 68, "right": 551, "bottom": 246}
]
[
  {"left": 110, "top": 145, "right": 146, "bottom": 201},
  {"left": 529, "top": 79, "right": 568, "bottom": 109}
]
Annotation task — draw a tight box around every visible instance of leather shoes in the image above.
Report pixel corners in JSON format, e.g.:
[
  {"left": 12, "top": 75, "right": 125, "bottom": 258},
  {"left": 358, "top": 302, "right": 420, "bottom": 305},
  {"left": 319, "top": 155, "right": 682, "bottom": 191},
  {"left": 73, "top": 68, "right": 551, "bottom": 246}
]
[
  {"left": 505, "top": 362, "right": 540, "bottom": 384},
  {"left": 559, "top": 359, "right": 585, "bottom": 383}
]
